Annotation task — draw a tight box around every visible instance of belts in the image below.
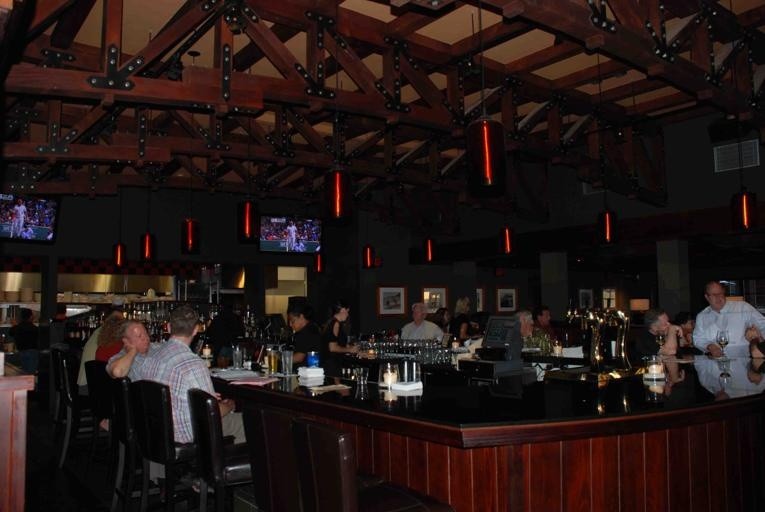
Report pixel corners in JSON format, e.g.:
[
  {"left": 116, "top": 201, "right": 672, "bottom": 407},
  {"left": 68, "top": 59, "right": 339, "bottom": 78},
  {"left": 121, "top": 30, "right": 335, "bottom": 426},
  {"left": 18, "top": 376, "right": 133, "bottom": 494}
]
[{"left": 175, "top": 441, "right": 196, "bottom": 448}]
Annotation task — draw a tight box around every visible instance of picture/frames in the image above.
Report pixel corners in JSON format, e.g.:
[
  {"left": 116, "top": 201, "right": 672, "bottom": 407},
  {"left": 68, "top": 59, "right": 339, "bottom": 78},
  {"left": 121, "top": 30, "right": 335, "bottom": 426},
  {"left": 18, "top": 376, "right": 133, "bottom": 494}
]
[
  {"left": 494, "top": 282, "right": 520, "bottom": 315},
  {"left": 474, "top": 286, "right": 486, "bottom": 315},
  {"left": 375, "top": 284, "right": 410, "bottom": 320},
  {"left": 419, "top": 284, "right": 448, "bottom": 320}
]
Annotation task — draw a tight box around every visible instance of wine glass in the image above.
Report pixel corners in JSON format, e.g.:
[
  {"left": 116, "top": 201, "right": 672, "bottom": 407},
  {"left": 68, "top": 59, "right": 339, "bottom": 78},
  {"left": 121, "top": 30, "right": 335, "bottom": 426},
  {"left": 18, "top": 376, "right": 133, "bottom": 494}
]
[
  {"left": 719, "top": 362, "right": 732, "bottom": 388},
  {"left": 715, "top": 330, "right": 731, "bottom": 362}
]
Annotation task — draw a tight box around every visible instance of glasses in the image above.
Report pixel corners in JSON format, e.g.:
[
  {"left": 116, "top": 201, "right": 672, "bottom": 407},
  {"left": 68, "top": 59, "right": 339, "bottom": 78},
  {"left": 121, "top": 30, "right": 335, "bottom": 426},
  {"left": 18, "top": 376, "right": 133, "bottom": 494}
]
[{"left": 707, "top": 292, "right": 725, "bottom": 298}]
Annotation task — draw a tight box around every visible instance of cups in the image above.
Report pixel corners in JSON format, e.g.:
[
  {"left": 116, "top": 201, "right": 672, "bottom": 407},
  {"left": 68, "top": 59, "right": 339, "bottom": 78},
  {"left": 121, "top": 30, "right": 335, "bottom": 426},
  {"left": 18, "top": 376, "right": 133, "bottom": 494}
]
[
  {"left": 643, "top": 381, "right": 666, "bottom": 402},
  {"left": 378, "top": 389, "right": 401, "bottom": 413},
  {"left": 355, "top": 384, "right": 370, "bottom": 401},
  {"left": 267, "top": 350, "right": 280, "bottom": 374},
  {"left": 282, "top": 350, "right": 294, "bottom": 376},
  {"left": 643, "top": 355, "right": 667, "bottom": 381},
  {"left": 354, "top": 368, "right": 371, "bottom": 387},
  {"left": 232, "top": 343, "right": 243, "bottom": 370},
  {"left": 377, "top": 362, "right": 401, "bottom": 391},
  {"left": 355, "top": 335, "right": 451, "bottom": 366}
]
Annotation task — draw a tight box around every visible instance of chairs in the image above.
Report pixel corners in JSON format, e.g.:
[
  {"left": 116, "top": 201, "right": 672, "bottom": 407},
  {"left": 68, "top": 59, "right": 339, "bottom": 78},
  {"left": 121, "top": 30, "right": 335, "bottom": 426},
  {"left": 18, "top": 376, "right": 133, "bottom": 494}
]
[{"left": 45, "top": 348, "right": 254, "bottom": 512}]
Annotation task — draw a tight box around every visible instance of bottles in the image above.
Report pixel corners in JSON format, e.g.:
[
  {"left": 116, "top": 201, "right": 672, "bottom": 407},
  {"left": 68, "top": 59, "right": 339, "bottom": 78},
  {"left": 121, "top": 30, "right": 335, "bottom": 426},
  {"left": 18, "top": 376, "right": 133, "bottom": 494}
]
[
  {"left": 67, "top": 326, "right": 100, "bottom": 352},
  {"left": 121, "top": 301, "right": 179, "bottom": 342},
  {"left": 340, "top": 367, "right": 357, "bottom": 382},
  {"left": 201, "top": 337, "right": 215, "bottom": 368},
  {"left": 452, "top": 336, "right": 461, "bottom": 352},
  {"left": 553, "top": 336, "right": 564, "bottom": 356},
  {"left": 242, "top": 304, "right": 264, "bottom": 340},
  {"left": 195, "top": 305, "right": 206, "bottom": 333},
  {"left": 206, "top": 303, "right": 224, "bottom": 328},
  {"left": 76, "top": 305, "right": 106, "bottom": 327}
]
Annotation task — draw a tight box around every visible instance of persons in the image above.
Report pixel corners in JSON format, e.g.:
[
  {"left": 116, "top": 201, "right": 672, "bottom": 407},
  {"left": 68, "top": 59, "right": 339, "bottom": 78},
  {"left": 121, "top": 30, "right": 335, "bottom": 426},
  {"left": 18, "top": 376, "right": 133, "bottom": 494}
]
[
  {"left": 106, "top": 321, "right": 159, "bottom": 382},
  {"left": 45, "top": 302, "right": 71, "bottom": 344},
  {"left": 0, "top": 198, "right": 55, "bottom": 240},
  {"left": 661, "top": 358, "right": 691, "bottom": 398},
  {"left": 130, "top": 306, "right": 246, "bottom": 446},
  {"left": 95, "top": 313, "right": 125, "bottom": 363},
  {"left": 400, "top": 301, "right": 444, "bottom": 341},
  {"left": 283, "top": 303, "right": 324, "bottom": 363},
  {"left": 694, "top": 359, "right": 765, "bottom": 401},
  {"left": 261, "top": 214, "right": 321, "bottom": 252},
  {"left": 204, "top": 296, "right": 245, "bottom": 368},
  {"left": 320, "top": 298, "right": 361, "bottom": 377},
  {"left": 8, "top": 308, "right": 41, "bottom": 376},
  {"left": 432, "top": 282, "right": 765, "bottom": 358},
  {"left": 75, "top": 306, "right": 124, "bottom": 395}
]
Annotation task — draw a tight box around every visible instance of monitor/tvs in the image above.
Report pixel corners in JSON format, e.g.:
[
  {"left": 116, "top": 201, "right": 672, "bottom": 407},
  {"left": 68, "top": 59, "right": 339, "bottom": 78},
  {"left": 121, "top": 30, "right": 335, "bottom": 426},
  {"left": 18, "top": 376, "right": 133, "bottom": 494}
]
[
  {"left": 0, "top": 193, "right": 61, "bottom": 246},
  {"left": 256, "top": 213, "right": 328, "bottom": 256}
]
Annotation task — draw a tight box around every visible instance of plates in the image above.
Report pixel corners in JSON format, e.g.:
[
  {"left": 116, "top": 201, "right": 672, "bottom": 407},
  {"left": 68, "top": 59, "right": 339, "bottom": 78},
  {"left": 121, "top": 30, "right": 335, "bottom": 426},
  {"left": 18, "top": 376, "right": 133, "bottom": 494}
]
[
  {"left": 56, "top": 287, "right": 175, "bottom": 304},
  {"left": 0, "top": 287, "right": 42, "bottom": 304}
]
